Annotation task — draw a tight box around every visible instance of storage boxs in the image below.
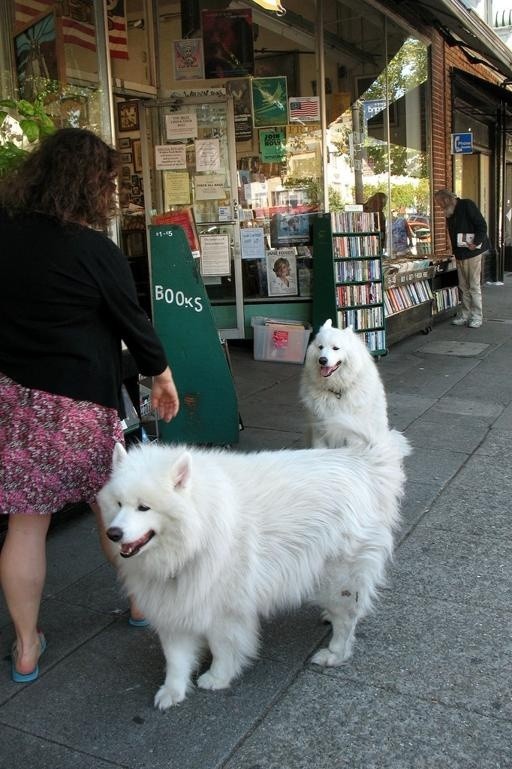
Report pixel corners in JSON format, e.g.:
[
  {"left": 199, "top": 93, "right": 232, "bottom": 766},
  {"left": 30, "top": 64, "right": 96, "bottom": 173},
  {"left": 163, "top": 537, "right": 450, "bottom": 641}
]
[{"left": 250, "top": 315, "right": 313, "bottom": 365}]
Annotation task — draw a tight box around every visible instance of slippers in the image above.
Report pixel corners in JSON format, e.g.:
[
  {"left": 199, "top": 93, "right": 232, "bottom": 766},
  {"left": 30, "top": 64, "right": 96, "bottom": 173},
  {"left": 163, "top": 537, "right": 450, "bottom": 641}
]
[
  {"left": 12, "top": 630, "right": 46, "bottom": 683},
  {"left": 129, "top": 618, "right": 149, "bottom": 627}
]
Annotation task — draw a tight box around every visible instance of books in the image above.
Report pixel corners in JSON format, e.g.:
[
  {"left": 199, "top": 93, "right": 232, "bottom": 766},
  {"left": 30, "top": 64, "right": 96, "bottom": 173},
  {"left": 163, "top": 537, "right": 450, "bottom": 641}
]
[
  {"left": 456, "top": 233, "right": 483, "bottom": 249},
  {"left": 330, "top": 211, "right": 460, "bottom": 352}
]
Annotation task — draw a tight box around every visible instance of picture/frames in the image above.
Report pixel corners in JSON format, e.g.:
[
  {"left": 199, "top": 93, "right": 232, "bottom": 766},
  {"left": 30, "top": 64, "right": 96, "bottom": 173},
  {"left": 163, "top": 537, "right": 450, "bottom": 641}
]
[
  {"left": 117, "top": 137, "right": 144, "bottom": 195},
  {"left": 9, "top": 4, "right": 68, "bottom": 107}
]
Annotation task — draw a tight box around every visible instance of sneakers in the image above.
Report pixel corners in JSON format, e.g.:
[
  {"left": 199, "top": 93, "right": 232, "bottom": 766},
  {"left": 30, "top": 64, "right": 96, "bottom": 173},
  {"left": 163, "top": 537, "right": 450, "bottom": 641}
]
[
  {"left": 452, "top": 319, "right": 467, "bottom": 326},
  {"left": 469, "top": 319, "right": 482, "bottom": 327}
]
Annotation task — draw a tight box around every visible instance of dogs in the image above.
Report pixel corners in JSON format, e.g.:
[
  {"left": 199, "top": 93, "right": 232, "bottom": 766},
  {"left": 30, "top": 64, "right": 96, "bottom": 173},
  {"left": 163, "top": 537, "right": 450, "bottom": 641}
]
[
  {"left": 296, "top": 317, "right": 392, "bottom": 450},
  {"left": 93, "top": 412, "right": 415, "bottom": 713}
]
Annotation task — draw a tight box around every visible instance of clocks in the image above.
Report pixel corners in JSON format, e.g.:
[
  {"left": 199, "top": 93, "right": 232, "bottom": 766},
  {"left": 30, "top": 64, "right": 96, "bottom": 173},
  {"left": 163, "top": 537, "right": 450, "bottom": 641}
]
[{"left": 117, "top": 101, "right": 140, "bottom": 132}]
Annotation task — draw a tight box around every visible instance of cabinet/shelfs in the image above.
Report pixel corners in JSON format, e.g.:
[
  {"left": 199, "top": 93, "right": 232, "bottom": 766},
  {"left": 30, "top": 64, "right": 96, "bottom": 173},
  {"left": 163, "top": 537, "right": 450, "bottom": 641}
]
[
  {"left": 381, "top": 254, "right": 463, "bottom": 347},
  {"left": 310, "top": 210, "right": 387, "bottom": 357}
]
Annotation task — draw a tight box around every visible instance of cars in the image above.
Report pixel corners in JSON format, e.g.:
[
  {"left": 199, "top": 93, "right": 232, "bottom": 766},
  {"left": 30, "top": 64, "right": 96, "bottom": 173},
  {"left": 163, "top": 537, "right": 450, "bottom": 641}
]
[{"left": 406, "top": 215, "right": 431, "bottom": 243}]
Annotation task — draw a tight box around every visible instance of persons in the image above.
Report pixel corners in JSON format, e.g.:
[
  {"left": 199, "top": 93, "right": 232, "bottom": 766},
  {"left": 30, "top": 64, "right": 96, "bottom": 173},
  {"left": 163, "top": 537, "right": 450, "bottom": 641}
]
[
  {"left": 0, "top": 129, "right": 183, "bottom": 683},
  {"left": 271, "top": 258, "right": 296, "bottom": 295},
  {"left": 434, "top": 189, "right": 490, "bottom": 328}
]
